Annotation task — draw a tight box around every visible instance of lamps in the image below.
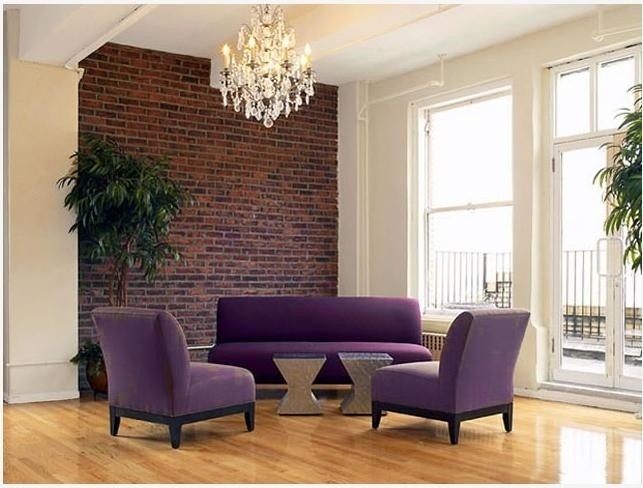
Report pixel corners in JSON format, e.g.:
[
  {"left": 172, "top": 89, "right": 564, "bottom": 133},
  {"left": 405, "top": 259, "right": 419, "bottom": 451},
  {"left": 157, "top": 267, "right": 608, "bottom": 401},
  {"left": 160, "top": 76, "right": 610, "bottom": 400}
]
[{"left": 218, "top": 4, "right": 319, "bottom": 129}]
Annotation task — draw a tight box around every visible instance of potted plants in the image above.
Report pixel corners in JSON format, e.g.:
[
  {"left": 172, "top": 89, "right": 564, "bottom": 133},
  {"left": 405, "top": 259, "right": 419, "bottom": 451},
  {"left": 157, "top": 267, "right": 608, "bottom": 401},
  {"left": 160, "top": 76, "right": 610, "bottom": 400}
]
[{"left": 56, "top": 134, "right": 200, "bottom": 401}]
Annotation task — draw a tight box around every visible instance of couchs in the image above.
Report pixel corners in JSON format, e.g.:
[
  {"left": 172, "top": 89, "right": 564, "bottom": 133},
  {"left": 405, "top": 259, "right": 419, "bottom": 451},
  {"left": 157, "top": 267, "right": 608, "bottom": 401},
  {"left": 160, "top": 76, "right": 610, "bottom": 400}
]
[
  {"left": 370, "top": 308, "right": 531, "bottom": 446},
  {"left": 208, "top": 294, "right": 432, "bottom": 386},
  {"left": 90, "top": 306, "right": 256, "bottom": 449}
]
